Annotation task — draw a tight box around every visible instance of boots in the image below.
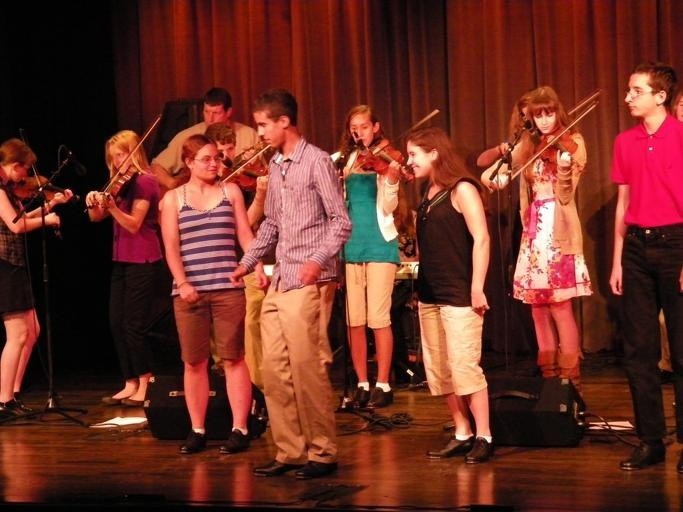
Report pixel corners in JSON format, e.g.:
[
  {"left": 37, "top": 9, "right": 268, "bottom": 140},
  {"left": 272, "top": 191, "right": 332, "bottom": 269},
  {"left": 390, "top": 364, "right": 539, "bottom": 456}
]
[
  {"left": 537, "top": 351, "right": 559, "bottom": 377},
  {"left": 557, "top": 348, "right": 585, "bottom": 392}
]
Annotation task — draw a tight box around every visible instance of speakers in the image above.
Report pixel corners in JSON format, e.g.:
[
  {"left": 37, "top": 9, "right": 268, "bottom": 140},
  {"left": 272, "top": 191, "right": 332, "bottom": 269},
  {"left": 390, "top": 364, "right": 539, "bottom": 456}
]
[
  {"left": 144, "top": 375, "right": 269, "bottom": 439},
  {"left": 473, "top": 377, "right": 591, "bottom": 447}
]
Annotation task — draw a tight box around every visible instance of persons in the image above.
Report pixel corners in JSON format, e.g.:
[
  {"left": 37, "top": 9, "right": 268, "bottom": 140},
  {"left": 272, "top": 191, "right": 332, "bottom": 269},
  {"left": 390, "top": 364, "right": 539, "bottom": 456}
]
[
  {"left": 85, "top": 130, "right": 164, "bottom": 408},
  {"left": 160, "top": 133, "right": 269, "bottom": 455},
  {"left": 229, "top": 89, "right": 351, "bottom": 479},
  {"left": 405, "top": 128, "right": 494, "bottom": 462},
  {"left": 476, "top": 86, "right": 593, "bottom": 394},
  {"left": 145, "top": 86, "right": 682, "bottom": 421},
  {"left": 0, "top": 138, "right": 73, "bottom": 419},
  {"left": 609, "top": 61, "right": 683, "bottom": 474}
]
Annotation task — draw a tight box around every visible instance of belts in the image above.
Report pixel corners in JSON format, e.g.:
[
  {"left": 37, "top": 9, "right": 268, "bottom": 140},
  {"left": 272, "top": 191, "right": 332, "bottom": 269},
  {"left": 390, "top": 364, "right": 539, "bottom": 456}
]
[{"left": 630, "top": 227, "right": 683, "bottom": 236}]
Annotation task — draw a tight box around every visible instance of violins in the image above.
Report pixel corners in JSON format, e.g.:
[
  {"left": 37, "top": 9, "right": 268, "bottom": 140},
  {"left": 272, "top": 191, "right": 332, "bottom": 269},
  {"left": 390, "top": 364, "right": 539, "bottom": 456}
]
[
  {"left": 356, "top": 139, "right": 414, "bottom": 180},
  {"left": 535, "top": 129, "right": 581, "bottom": 175},
  {"left": 102, "top": 164, "right": 137, "bottom": 209},
  {"left": 10, "top": 174, "right": 79, "bottom": 202},
  {"left": 218, "top": 157, "right": 270, "bottom": 192}
]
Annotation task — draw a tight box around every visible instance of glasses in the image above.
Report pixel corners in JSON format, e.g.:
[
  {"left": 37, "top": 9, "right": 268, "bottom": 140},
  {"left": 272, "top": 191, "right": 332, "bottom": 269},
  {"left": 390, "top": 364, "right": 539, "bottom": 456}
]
[
  {"left": 624, "top": 88, "right": 660, "bottom": 100},
  {"left": 193, "top": 157, "right": 221, "bottom": 164}
]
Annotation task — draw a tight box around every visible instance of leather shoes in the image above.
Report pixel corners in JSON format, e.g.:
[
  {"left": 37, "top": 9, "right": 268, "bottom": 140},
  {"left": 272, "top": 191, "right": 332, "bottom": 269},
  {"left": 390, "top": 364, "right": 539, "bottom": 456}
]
[
  {"left": 102, "top": 397, "right": 131, "bottom": 405},
  {"left": 121, "top": 396, "right": 144, "bottom": 406},
  {"left": 620, "top": 440, "right": 666, "bottom": 470},
  {"left": 251, "top": 460, "right": 336, "bottom": 476},
  {"left": 427, "top": 436, "right": 495, "bottom": 460},
  {"left": 219, "top": 429, "right": 250, "bottom": 454},
  {"left": 0, "top": 399, "right": 36, "bottom": 422},
  {"left": 14, "top": 392, "right": 35, "bottom": 414},
  {"left": 347, "top": 387, "right": 395, "bottom": 409},
  {"left": 179, "top": 431, "right": 208, "bottom": 453}
]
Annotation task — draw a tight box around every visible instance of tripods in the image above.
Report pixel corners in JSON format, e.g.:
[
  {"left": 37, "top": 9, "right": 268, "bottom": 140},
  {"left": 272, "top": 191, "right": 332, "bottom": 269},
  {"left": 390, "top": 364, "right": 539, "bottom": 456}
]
[
  {"left": 324, "top": 147, "right": 390, "bottom": 431},
  {"left": 0, "top": 162, "right": 94, "bottom": 430}
]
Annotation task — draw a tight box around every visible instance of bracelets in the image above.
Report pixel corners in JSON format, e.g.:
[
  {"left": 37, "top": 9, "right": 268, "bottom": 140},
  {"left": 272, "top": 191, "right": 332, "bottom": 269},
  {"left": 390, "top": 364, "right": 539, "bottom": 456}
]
[{"left": 176, "top": 280, "right": 191, "bottom": 290}]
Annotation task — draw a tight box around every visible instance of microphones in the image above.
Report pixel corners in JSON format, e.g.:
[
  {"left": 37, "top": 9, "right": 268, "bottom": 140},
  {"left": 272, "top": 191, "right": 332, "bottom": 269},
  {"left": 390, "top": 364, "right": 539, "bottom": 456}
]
[
  {"left": 353, "top": 131, "right": 370, "bottom": 156},
  {"left": 62, "top": 143, "right": 88, "bottom": 178},
  {"left": 516, "top": 104, "right": 532, "bottom": 129},
  {"left": 218, "top": 150, "right": 233, "bottom": 168}
]
